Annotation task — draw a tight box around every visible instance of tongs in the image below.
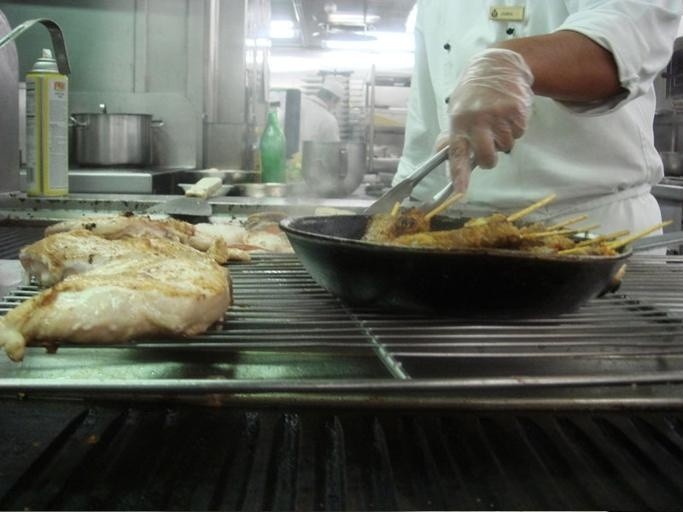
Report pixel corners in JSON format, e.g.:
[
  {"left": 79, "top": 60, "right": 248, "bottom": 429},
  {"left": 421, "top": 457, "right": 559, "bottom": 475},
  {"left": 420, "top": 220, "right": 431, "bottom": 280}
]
[{"left": 362, "top": 146, "right": 478, "bottom": 216}]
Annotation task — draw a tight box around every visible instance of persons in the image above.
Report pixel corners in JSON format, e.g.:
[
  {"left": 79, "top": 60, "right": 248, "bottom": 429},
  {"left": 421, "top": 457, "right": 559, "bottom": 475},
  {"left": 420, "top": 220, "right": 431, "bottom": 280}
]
[
  {"left": 392, "top": 0, "right": 683, "bottom": 255},
  {"left": 269, "top": 80, "right": 345, "bottom": 152}
]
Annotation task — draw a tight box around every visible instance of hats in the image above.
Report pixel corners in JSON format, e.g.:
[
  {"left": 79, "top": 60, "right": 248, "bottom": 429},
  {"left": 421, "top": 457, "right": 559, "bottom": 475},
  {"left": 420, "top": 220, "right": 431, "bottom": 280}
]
[{"left": 322, "top": 79, "right": 345, "bottom": 99}]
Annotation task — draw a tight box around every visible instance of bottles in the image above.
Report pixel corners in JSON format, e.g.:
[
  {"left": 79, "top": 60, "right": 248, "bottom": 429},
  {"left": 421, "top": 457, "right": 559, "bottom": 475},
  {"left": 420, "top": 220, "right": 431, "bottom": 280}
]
[{"left": 260, "top": 110, "right": 286, "bottom": 183}]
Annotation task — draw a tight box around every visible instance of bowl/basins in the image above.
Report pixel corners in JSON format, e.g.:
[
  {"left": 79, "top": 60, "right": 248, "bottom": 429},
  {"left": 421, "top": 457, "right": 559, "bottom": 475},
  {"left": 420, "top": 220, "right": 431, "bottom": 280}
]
[
  {"left": 184, "top": 169, "right": 259, "bottom": 196},
  {"left": 231, "top": 183, "right": 294, "bottom": 197},
  {"left": 656, "top": 151, "right": 683, "bottom": 177}
]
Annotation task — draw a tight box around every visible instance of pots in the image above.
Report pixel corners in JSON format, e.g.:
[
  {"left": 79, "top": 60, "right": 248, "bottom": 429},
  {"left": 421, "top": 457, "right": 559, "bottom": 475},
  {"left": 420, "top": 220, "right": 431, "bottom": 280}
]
[
  {"left": 278, "top": 212, "right": 683, "bottom": 315},
  {"left": 301, "top": 140, "right": 367, "bottom": 200},
  {"left": 69, "top": 112, "right": 165, "bottom": 169}
]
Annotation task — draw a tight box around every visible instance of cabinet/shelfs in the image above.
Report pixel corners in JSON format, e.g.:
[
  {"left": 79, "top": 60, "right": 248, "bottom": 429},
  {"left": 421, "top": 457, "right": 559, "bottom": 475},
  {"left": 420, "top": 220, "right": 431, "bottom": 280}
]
[{"left": 363, "top": 64, "right": 410, "bottom": 176}]
